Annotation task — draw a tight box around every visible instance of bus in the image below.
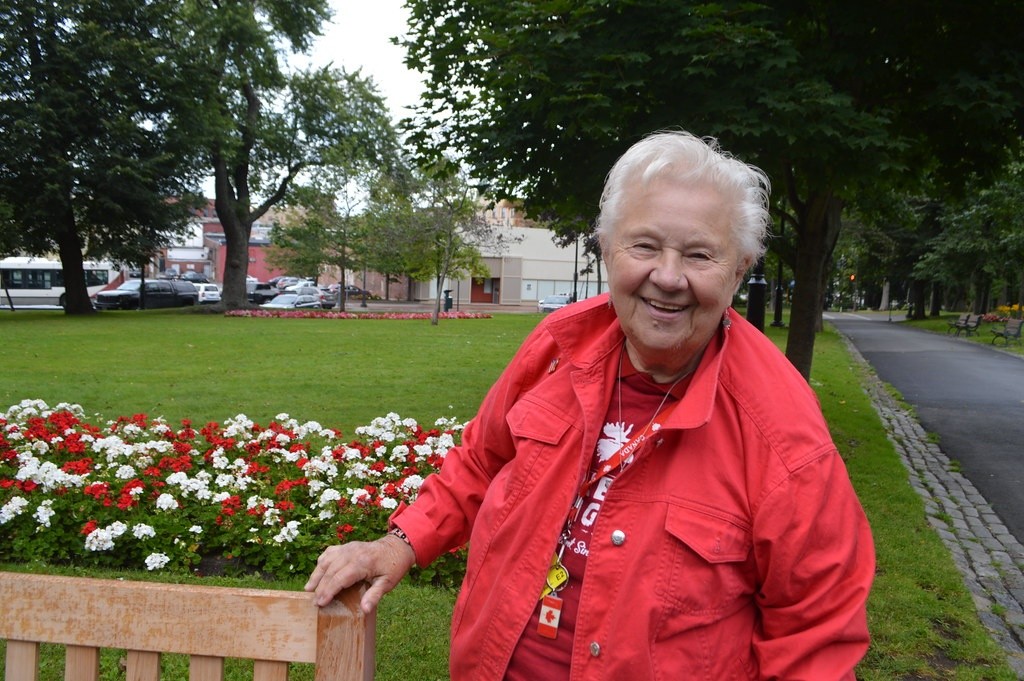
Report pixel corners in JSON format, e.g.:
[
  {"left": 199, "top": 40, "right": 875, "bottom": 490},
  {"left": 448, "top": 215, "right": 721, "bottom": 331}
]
[{"left": 0, "top": 256, "right": 122, "bottom": 308}]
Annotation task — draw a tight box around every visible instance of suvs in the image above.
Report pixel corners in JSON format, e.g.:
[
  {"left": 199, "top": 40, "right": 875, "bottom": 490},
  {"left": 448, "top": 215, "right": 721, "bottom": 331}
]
[{"left": 93, "top": 279, "right": 199, "bottom": 310}]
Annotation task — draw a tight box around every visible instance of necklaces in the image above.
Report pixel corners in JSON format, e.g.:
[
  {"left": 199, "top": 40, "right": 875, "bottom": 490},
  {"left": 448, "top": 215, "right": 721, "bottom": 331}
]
[{"left": 618, "top": 342, "right": 696, "bottom": 474}]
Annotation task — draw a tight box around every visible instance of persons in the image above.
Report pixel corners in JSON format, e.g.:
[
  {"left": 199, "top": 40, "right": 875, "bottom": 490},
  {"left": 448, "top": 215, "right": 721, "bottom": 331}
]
[{"left": 304, "top": 128, "right": 876, "bottom": 681}]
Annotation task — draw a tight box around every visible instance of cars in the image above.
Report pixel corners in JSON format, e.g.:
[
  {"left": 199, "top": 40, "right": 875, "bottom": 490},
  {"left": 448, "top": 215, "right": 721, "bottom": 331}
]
[
  {"left": 537, "top": 292, "right": 583, "bottom": 313},
  {"left": 129, "top": 266, "right": 370, "bottom": 311}
]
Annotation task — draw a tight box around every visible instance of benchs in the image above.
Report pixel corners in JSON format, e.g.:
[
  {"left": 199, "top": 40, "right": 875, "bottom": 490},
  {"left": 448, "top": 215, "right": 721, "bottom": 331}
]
[
  {"left": 990, "top": 318, "right": 1024, "bottom": 346},
  {"left": 956, "top": 314, "right": 981, "bottom": 338},
  {"left": 947, "top": 313, "right": 970, "bottom": 337}
]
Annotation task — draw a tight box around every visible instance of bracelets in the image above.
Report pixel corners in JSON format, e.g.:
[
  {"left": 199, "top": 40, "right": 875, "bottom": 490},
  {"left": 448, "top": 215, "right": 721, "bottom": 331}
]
[{"left": 389, "top": 527, "right": 411, "bottom": 545}]
